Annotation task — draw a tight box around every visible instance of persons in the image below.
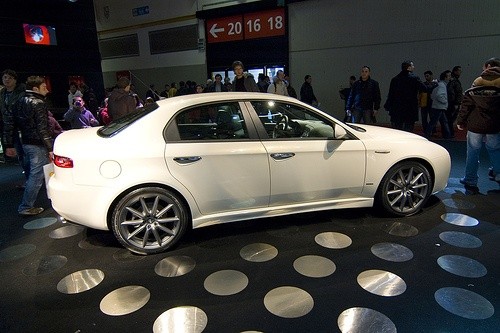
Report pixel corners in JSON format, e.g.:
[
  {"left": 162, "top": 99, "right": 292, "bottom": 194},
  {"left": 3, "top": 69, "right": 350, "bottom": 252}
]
[
  {"left": 232, "top": 60, "right": 263, "bottom": 104},
  {"left": 63, "top": 81, "right": 144, "bottom": 129},
  {"left": 456, "top": 57, "right": 500, "bottom": 185},
  {"left": 346, "top": 65, "right": 381, "bottom": 124},
  {"left": 344, "top": 76, "right": 357, "bottom": 123},
  {"left": 417, "top": 70, "right": 439, "bottom": 136},
  {"left": 300, "top": 75, "right": 318, "bottom": 105},
  {"left": 384, "top": 60, "right": 438, "bottom": 133},
  {"left": 143, "top": 70, "right": 297, "bottom": 106},
  {"left": 0, "top": 69, "right": 64, "bottom": 215},
  {"left": 109, "top": 77, "right": 136, "bottom": 120},
  {"left": 422, "top": 69, "right": 451, "bottom": 141},
  {"left": 446, "top": 66, "right": 463, "bottom": 136}
]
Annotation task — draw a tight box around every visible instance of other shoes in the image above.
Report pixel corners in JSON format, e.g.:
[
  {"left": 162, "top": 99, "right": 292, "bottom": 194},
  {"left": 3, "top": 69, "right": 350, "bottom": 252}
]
[
  {"left": 16, "top": 183, "right": 25, "bottom": 188},
  {"left": 488, "top": 168, "right": 500, "bottom": 181},
  {"left": 460, "top": 178, "right": 477, "bottom": 186}
]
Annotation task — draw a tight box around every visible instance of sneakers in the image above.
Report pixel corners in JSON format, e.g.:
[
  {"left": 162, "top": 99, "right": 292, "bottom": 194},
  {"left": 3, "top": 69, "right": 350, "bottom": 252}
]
[{"left": 19, "top": 207, "right": 44, "bottom": 214}]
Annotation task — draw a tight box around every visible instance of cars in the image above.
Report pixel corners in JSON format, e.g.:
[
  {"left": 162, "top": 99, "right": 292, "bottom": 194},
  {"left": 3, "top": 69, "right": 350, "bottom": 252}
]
[{"left": 42, "top": 91, "right": 451, "bottom": 256}]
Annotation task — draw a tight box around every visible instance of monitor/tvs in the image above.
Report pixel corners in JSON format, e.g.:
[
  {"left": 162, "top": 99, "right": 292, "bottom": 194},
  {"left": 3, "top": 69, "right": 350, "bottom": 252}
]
[{"left": 23, "top": 24, "right": 58, "bottom": 46}]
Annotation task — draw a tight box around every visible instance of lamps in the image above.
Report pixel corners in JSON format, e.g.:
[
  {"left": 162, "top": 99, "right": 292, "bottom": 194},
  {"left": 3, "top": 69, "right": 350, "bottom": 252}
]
[{"left": 203, "top": 1, "right": 243, "bottom": 10}]
